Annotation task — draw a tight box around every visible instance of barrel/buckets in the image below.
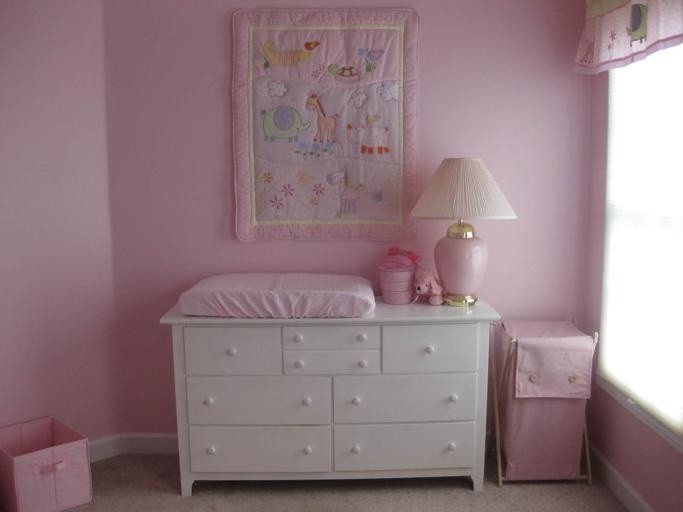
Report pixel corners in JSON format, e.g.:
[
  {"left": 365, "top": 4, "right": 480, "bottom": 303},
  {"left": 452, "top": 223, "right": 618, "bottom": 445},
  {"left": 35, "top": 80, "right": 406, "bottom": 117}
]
[{"left": 377, "top": 262, "right": 417, "bottom": 305}]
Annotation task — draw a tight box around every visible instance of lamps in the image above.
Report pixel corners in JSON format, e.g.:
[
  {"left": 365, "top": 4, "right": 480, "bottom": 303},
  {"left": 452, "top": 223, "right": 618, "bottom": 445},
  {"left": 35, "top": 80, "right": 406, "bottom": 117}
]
[{"left": 407, "top": 158, "right": 517, "bottom": 305}]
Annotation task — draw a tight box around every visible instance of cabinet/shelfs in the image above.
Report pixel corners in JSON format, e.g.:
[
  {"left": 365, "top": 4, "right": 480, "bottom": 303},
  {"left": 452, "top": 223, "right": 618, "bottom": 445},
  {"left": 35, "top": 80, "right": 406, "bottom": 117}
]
[{"left": 159, "top": 294, "right": 501, "bottom": 496}]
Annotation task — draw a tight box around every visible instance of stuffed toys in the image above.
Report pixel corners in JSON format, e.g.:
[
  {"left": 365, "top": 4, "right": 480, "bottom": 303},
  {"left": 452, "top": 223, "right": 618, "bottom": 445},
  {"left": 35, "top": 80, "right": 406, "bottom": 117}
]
[{"left": 413, "top": 268, "right": 445, "bottom": 305}]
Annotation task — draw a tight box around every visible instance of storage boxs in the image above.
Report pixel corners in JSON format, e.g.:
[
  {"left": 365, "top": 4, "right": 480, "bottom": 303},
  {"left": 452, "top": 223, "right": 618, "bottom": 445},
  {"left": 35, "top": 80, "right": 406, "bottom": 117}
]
[{"left": 0, "top": 417, "right": 92, "bottom": 510}]
